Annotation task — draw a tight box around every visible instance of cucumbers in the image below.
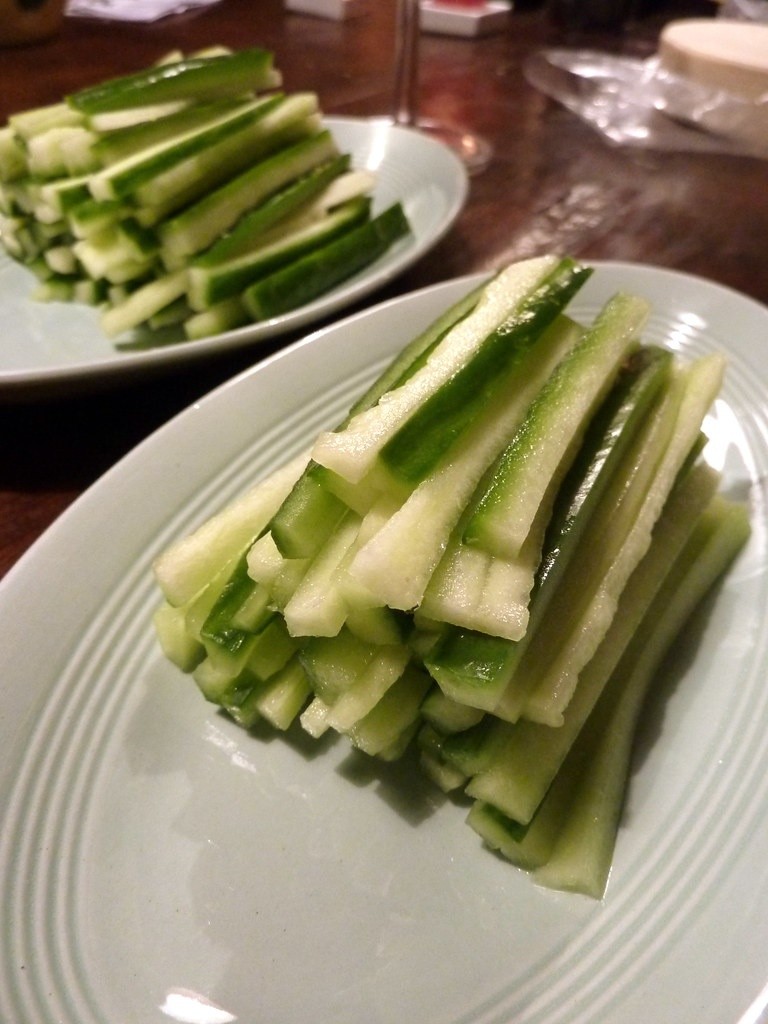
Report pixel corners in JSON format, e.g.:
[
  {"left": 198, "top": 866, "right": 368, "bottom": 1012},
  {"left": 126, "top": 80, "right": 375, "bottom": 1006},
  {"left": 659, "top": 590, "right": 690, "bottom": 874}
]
[
  {"left": 0, "top": 44, "right": 413, "bottom": 351},
  {"left": 152, "top": 252, "right": 748, "bottom": 902}
]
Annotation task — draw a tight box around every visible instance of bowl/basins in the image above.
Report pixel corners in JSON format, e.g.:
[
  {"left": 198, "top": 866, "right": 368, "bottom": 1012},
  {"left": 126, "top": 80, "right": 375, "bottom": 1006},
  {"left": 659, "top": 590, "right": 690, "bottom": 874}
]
[{"left": 656, "top": 19, "right": 768, "bottom": 142}]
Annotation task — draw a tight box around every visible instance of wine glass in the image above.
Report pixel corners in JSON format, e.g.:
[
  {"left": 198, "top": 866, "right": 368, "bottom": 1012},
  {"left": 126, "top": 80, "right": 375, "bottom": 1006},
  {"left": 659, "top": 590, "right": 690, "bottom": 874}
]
[{"left": 353, "top": 0, "right": 496, "bottom": 176}]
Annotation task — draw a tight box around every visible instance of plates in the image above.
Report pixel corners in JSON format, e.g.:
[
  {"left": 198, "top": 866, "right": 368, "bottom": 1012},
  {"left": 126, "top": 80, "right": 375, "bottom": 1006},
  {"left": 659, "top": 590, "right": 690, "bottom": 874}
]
[
  {"left": 0, "top": 258, "right": 768, "bottom": 1024},
  {"left": 0, "top": 117, "right": 471, "bottom": 385}
]
